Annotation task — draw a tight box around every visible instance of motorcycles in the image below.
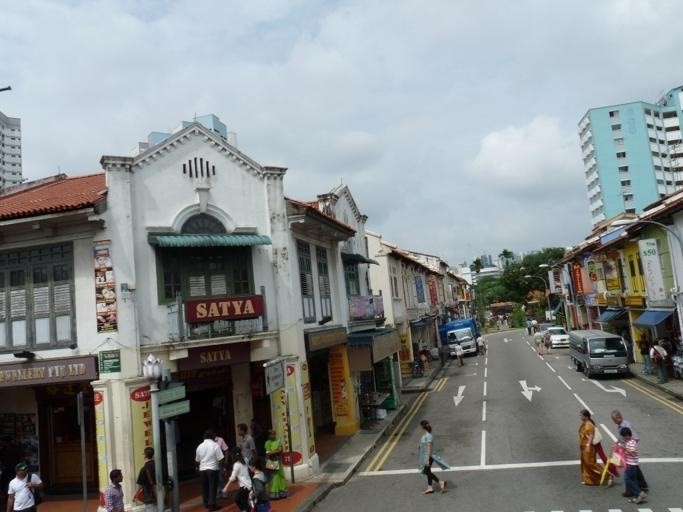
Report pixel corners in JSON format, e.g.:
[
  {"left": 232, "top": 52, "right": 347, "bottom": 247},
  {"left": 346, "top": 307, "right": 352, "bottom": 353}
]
[{"left": 409, "top": 357, "right": 424, "bottom": 379}]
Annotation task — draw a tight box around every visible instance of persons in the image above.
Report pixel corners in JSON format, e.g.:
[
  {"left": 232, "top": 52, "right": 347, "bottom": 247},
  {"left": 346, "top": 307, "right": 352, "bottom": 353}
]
[
  {"left": 0, "top": 437, "right": 20, "bottom": 492},
  {"left": 476, "top": 334, "right": 485, "bottom": 354},
  {"left": 526, "top": 318, "right": 552, "bottom": 355},
  {"left": 103, "top": 469, "right": 124, "bottom": 512},
  {"left": 136, "top": 447, "right": 156, "bottom": 512},
  {"left": 578, "top": 409, "right": 613, "bottom": 487},
  {"left": 638, "top": 335, "right": 652, "bottom": 374},
  {"left": 6, "top": 463, "right": 44, "bottom": 512},
  {"left": 650, "top": 341, "right": 668, "bottom": 384},
  {"left": 618, "top": 428, "right": 647, "bottom": 504},
  {"left": 455, "top": 342, "right": 464, "bottom": 366},
  {"left": 418, "top": 421, "right": 450, "bottom": 494},
  {"left": 195, "top": 419, "right": 288, "bottom": 512},
  {"left": 611, "top": 410, "right": 648, "bottom": 497}
]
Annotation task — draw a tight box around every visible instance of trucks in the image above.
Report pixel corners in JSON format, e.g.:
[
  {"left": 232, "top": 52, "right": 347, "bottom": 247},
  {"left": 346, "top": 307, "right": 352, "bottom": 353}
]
[
  {"left": 439, "top": 318, "right": 480, "bottom": 356},
  {"left": 567, "top": 329, "right": 628, "bottom": 378}
]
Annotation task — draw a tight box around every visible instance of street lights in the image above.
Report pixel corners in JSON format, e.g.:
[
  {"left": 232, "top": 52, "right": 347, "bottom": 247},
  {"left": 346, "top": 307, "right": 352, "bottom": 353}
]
[
  {"left": 538, "top": 264, "right": 578, "bottom": 329},
  {"left": 524, "top": 274, "right": 552, "bottom": 321},
  {"left": 141, "top": 353, "right": 165, "bottom": 511},
  {"left": 609, "top": 219, "right": 682, "bottom": 261}
]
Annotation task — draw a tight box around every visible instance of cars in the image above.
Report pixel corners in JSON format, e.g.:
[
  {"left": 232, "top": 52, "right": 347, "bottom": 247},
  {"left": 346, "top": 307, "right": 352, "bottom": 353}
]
[
  {"left": 535, "top": 324, "right": 556, "bottom": 342},
  {"left": 543, "top": 326, "right": 570, "bottom": 349}
]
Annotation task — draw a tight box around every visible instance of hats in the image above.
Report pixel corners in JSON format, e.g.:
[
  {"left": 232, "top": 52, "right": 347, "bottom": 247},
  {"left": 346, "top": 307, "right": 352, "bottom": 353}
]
[{"left": 14, "top": 462, "right": 30, "bottom": 473}]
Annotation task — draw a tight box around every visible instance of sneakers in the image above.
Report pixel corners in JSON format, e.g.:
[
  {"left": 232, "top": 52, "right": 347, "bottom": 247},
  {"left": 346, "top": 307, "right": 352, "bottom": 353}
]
[
  {"left": 422, "top": 488, "right": 435, "bottom": 495},
  {"left": 635, "top": 491, "right": 648, "bottom": 504},
  {"left": 440, "top": 480, "right": 447, "bottom": 494}
]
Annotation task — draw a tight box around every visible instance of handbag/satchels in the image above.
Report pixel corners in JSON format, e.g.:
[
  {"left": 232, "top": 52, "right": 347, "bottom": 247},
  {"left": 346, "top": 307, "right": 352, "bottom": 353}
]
[
  {"left": 265, "top": 459, "right": 280, "bottom": 471},
  {"left": 149, "top": 483, "right": 170, "bottom": 506},
  {"left": 585, "top": 426, "right": 603, "bottom": 446},
  {"left": 27, "top": 472, "right": 45, "bottom": 507}
]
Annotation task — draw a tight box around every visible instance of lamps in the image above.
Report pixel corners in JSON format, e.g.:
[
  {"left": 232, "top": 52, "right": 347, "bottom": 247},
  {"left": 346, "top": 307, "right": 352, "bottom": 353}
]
[{"left": 12, "top": 346, "right": 34, "bottom": 361}]
